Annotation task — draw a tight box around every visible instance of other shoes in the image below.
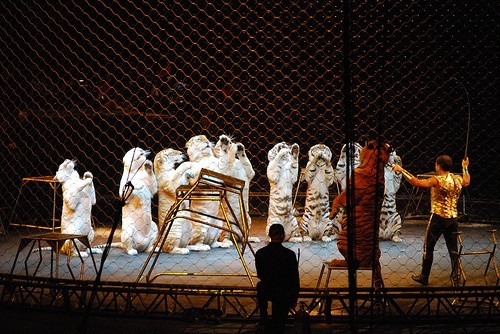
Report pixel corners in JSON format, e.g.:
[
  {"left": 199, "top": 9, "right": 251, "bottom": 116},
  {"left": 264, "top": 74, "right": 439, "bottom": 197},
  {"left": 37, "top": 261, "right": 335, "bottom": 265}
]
[{"left": 412, "top": 274, "right": 428, "bottom": 286}]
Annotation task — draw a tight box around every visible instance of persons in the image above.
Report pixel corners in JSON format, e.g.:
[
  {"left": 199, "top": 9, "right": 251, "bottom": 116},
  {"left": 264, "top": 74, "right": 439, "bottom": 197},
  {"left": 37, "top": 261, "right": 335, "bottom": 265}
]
[
  {"left": 255, "top": 224, "right": 300, "bottom": 334},
  {"left": 394, "top": 157, "right": 470, "bottom": 287}
]
